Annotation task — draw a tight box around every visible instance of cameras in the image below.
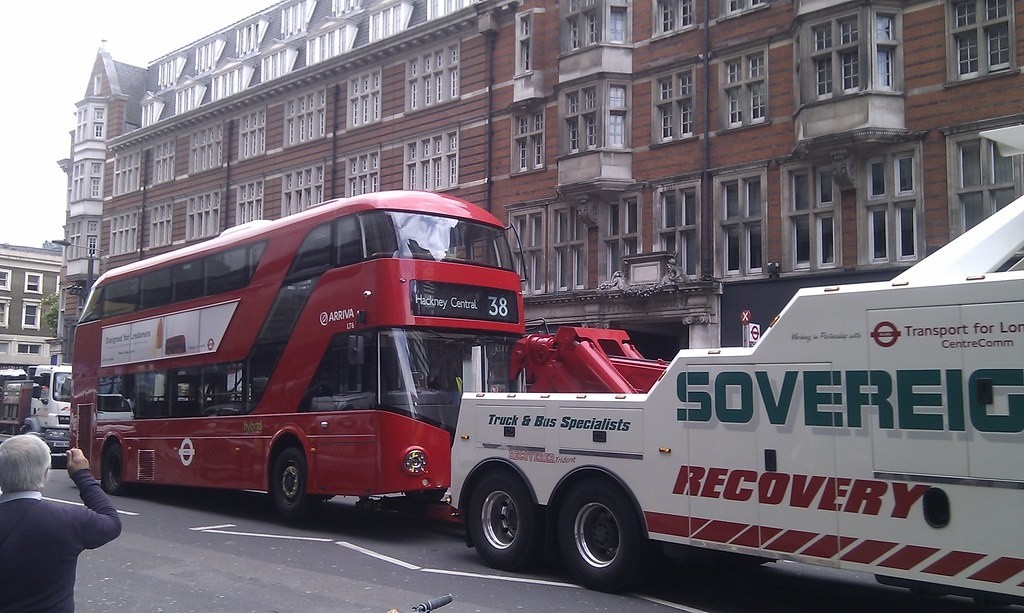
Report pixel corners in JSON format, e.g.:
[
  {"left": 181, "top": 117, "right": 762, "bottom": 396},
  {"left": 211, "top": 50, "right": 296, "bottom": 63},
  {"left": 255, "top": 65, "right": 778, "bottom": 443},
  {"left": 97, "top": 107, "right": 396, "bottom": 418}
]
[{"left": 51, "top": 453, "right": 68, "bottom": 469}]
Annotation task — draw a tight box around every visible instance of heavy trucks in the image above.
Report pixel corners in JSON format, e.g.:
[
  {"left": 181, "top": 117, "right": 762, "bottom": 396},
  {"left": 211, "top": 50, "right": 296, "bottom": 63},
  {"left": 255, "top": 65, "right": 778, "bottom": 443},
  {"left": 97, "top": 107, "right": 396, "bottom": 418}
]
[{"left": 1, "top": 364, "right": 134, "bottom": 469}]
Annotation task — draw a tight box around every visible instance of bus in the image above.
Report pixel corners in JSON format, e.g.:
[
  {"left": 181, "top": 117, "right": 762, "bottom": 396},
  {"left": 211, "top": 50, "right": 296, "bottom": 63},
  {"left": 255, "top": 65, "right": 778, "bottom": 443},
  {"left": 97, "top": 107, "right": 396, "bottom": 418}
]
[{"left": 68, "top": 190, "right": 550, "bottom": 524}]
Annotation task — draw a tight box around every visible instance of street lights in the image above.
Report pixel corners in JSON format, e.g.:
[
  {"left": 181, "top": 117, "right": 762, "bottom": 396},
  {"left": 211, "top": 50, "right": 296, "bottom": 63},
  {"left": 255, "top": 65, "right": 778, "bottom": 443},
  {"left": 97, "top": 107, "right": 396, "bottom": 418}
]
[{"left": 51, "top": 239, "right": 104, "bottom": 302}]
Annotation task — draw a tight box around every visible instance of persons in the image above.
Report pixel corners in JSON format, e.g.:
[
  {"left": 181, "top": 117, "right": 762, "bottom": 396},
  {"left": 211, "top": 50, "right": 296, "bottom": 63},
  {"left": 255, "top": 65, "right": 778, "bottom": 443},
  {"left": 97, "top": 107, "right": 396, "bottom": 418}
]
[{"left": 0, "top": 434, "right": 122, "bottom": 613}]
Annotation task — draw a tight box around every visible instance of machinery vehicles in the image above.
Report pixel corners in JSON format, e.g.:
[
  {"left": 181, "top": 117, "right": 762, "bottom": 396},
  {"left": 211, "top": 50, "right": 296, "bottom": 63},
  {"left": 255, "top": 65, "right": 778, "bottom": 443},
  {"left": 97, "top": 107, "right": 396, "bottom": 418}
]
[{"left": 440, "top": 195, "right": 1024, "bottom": 613}]
